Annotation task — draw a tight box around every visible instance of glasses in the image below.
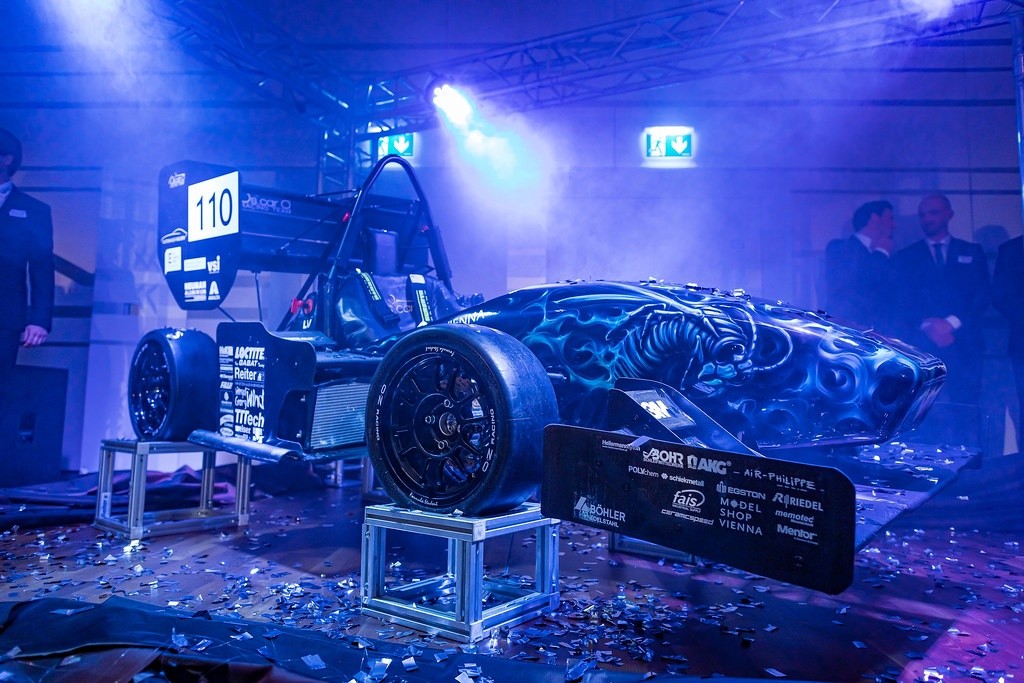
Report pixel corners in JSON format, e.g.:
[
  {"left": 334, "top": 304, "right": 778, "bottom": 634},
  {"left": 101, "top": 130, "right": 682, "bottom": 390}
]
[{"left": 919, "top": 209, "right": 946, "bottom": 218}]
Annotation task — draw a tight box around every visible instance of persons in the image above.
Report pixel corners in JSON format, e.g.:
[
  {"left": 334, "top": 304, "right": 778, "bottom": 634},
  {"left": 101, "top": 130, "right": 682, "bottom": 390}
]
[
  {"left": 993, "top": 234, "right": 1024, "bottom": 482},
  {"left": 814, "top": 199, "right": 913, "bottom": 346},
  {"left": 893, "top": 193, "right": 990, "bottom": 500},
  {"left": 0, "top": 127, "right": 56, "bottom": 407}
]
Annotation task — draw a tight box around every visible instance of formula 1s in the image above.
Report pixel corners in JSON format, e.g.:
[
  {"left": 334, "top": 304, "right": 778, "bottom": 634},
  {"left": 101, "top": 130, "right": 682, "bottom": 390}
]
[{"left": 122, "top": 149, "right": 949, "bottom": 521}]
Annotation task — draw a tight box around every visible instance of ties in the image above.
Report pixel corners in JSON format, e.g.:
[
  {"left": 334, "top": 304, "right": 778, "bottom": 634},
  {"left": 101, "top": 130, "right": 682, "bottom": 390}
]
[{"left": 932, "top": 242, "right": 945, "bottom": 276}]
